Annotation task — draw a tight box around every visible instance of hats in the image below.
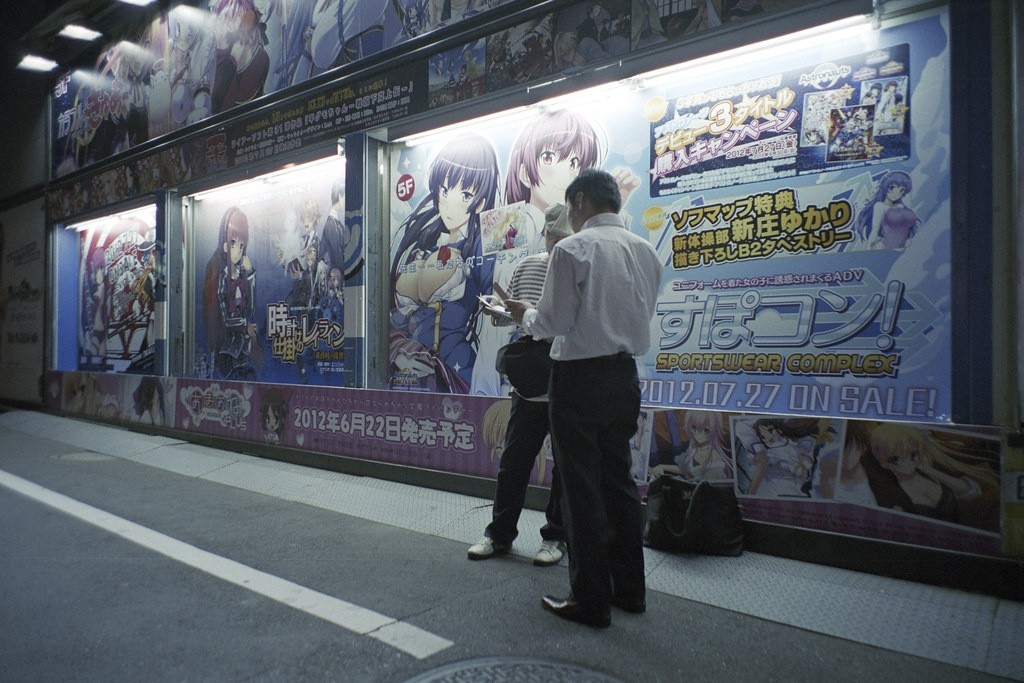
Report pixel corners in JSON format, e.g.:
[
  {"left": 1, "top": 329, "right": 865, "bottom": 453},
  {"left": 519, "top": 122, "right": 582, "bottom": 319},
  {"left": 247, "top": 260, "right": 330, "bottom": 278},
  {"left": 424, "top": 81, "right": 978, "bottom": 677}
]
[{"left": 544, "top": 203, "right": 574, "bottom": 237}]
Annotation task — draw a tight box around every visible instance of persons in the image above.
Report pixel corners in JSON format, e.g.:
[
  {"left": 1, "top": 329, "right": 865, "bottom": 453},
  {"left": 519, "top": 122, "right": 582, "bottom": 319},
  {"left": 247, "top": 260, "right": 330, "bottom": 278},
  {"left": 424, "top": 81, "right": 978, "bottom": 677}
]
[
  {"left": 501, "top": 165, "right": 663, "bottom": 629},
  {"left": 466, "top": 201, "right": 575, "bottom": 568}
]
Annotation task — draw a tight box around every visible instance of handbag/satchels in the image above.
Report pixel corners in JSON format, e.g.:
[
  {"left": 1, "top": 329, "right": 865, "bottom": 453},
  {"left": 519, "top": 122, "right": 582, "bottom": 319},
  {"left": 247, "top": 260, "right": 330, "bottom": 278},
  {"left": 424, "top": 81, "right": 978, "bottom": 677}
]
[
  {"left": 496, "top": 335, "right": 554, "bottom": 398},
  {"left": 643, "top": 472, "right": 744, "bottom": 556}
]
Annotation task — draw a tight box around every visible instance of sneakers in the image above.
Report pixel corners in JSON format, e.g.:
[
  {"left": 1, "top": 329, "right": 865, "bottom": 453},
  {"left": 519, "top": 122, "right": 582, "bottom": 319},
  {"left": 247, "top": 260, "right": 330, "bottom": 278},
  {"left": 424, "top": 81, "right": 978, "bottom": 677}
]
[
  {"left": 468, "top": 537, "right": 513, "bottom": 558},
  {"left": 534, "top": 539, "right": 567, "bottom": 565}
]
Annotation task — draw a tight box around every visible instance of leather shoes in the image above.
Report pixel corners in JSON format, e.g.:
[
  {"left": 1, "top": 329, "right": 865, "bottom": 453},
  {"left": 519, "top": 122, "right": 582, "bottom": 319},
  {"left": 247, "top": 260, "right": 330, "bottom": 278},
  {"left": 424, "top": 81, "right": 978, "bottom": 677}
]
[
  {"left": 542, "top": 593, "right": 611, "bottom": 628},
  {"left": 609, "top": 595, "right": 646, "bottom": 614}
]
